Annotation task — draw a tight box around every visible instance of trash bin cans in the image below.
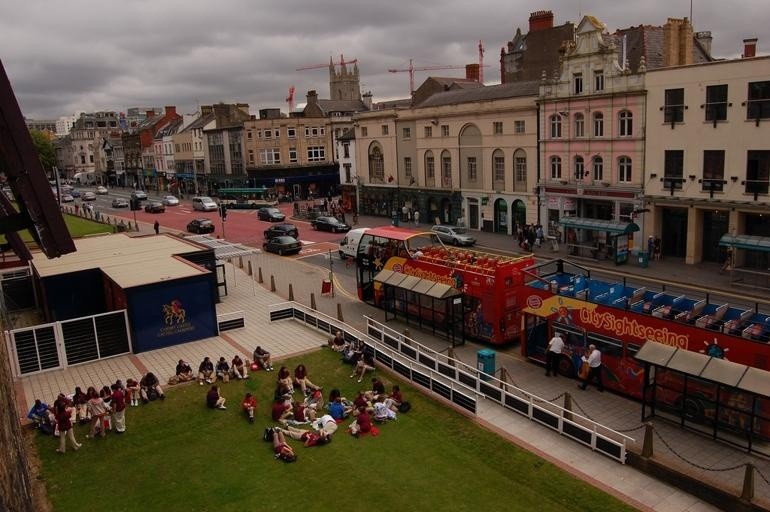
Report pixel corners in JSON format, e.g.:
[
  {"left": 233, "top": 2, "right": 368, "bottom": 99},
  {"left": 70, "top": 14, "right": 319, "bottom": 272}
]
[
  {"left": 392, "top": 218, "right": 400, "bottom": 226},
  {"left": 477, "top": 348, "right": 496, "bottom": 381},
  {"left": 638, "top": 251, "right": 649, "bottom": 268}
]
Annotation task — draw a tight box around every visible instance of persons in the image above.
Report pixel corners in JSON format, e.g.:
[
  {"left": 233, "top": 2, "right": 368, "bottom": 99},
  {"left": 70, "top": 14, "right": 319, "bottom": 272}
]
[
  {"left": 332, "top": 332, "right": 375, "bottom": 383},
  {"left": 254, "top": 346, "right": 274, "bottom": 371},
  {"left": 648, "top": 235, "right": 662, "bottom": 261},
  {"left": 199, "top": 357, "right": 217, "bottom": 385},
  {"left": 27, "top": 372, "right": 166, "bottom": 453},
  {"left": 154, "top": 220, "right": 159, "bottom": 234},
  {"left": 243, "top": 393, "right": 257, "bottom": 418},
  {"left": 216, "top": 357, "right": 229, "bottom": 382},
  {"left": 75, "top": 203, "right": 95, "bottom": 214},
  {"left": 544, "top": 335, "right": 565, "bottom": 377},
  {"left": 264, "top": 365, "right": 352, "bottom": 463},
  {"left": 207, "top": 385, "right": 226, "bottom": 409},
  {"left": 411, "top": 248, "right": 423, "bottom": 259},
  {"left": 577, "top": 345, "right": 604, "bottom": 393},
  {"left": 231, "top": 355, "right": 248, "bottom": 379},
  {"left": 346, "top": 378, "right": 403, "bottom": 437},
  {"left": 515, "top": 222, "right": 543, "bottom": 253},
  {"left": 175, "top": 360, "right": 193, "bottom": 381},
  {"left": 270, "top": 191, "right": 419, "bottom": 226}
]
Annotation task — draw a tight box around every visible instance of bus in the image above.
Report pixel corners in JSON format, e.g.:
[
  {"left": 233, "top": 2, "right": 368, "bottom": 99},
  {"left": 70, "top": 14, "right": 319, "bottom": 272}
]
[
  {"left": 356, "top": 226, "right": 536, "bottom": 348},
  {"left": 519, "top": 257, "right": 770, "bottom": 448},
  {"left": 215, "top": 187, "right": 279, "bottom": 208}
]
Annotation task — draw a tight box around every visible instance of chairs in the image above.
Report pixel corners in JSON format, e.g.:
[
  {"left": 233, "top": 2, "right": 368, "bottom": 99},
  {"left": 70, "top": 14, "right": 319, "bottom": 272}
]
[
  {"left": 549, "top": 272, "right": 770, "bottom": 343},
  {"left": 400, "top": 245, "right": 499, "bottom": 275}
]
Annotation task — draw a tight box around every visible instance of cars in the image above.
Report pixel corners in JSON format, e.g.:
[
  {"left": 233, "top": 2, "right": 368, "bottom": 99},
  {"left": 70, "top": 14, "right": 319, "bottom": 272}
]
[
  {"left": 82, "top": 192, "right": 97, "bottom": 200},
  {"left": 431, "top": 225, "right": 478, "bottom": 247},
  {"left": 95, "top": 186, "right": 108, "bottom": 195},
  {"left": 131, "top": 191, "right": 148, "bottom": 200},
  {"left": 311, "top": 216, "right": 351, "bottom": 234},
  {"left": 112, "top": 197, "right": 129, "bottom": 208},
  {"left": 52, "top": 184, "right": 81, "bottom": 203},
  {"left": 3, "top": 187, "right": 14, "bottom": 200},
  {"left": 263, "top": 236, "right": 301, "bottom": 255}
]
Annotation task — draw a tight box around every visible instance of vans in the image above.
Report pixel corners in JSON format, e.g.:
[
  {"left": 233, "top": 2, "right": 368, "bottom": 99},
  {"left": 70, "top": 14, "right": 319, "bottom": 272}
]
[
  {"left": 338, "top": 229, "right": 371, "bottom": 259},
  {"left": 192, "top": 195, "right": 218, "bottom": 213}
]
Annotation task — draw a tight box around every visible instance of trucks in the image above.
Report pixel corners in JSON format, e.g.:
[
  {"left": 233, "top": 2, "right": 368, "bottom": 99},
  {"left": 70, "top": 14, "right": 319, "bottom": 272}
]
[{"left": 69, "top": 172, "right": 96, "bottom": 185}]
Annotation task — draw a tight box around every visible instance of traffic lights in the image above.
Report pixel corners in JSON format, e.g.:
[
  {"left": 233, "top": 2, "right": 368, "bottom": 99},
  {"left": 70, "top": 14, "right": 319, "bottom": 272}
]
[
  {"left": 136, "top": 199, "right": 142, "bottom": 210},
  {"left": 223, "top": 206, "right": 228, "bottom": 217}
]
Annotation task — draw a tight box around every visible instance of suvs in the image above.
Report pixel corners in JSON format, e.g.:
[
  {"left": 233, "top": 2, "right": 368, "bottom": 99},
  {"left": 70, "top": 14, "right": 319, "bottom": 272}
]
[
  {"left": 264, "top": 224, "right": 299, "bottom": 241},
  {"left": 49, "top": 177, "right": 57, "bottom": 186},
  {"left": 257, "top": 208, "right": 286, "bottom": 221},
  {"left": 187, "top": 217, "right": 215, "bottom": 234}
]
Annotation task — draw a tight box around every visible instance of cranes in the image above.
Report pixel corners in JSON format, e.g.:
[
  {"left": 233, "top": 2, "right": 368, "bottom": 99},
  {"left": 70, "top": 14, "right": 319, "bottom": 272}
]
[
  {"left": 284, "top": 85, "right": 294, "bottom": 115},
  {"left": 293, "top": 59, "right": 357, "bottom": 73},
  {"left": 478, "top": 40, "right": 488, "bottom": 84},
  {"left": 388, "top": 58, "right": 490, "bottom": 94}
]
